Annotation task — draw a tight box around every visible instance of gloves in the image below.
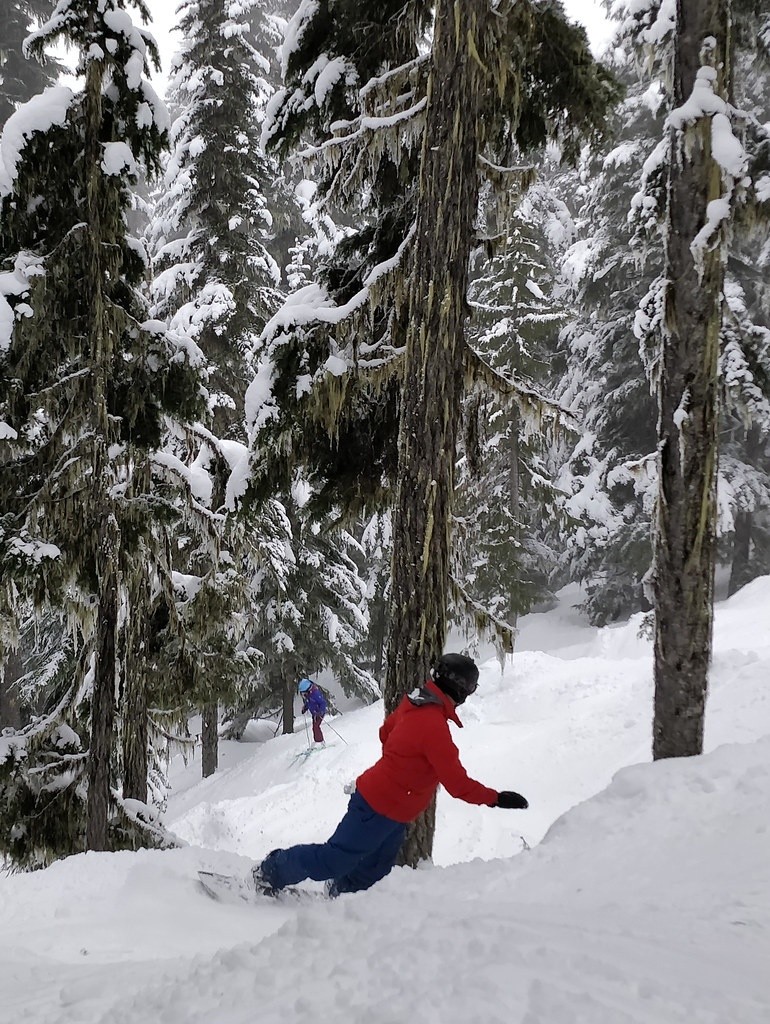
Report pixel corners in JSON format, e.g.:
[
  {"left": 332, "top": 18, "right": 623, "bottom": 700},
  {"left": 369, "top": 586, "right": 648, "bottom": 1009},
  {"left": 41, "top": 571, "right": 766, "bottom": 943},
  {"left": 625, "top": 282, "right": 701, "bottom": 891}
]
[
  {"left": 316, "top": 711, "right": 322, "bottom": 717},
  {"left": 302, "top": 708, "right": 306, "bottom": 715},
  {"left": 497, "top": 791, "right": 528, "bottom": 808}
]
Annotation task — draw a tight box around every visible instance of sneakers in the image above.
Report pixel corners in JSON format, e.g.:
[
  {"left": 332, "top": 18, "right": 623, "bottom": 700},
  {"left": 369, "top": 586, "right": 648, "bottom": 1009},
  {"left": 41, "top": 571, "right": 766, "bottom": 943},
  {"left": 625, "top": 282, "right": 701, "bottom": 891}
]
[
  {"left": 323, "top": 878, "right": 339, "bottom": 901},
  {"left": 252, "top": 865, "right": 278, "bottom": 901}
]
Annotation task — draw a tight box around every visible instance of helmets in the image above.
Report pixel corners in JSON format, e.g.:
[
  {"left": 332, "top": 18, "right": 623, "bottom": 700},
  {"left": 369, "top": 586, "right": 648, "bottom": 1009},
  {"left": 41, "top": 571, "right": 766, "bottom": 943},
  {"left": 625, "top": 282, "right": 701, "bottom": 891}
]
[
  {"left": 298, "top": 679, "right": 311, "bottom": 692},
  {"left": 434, "top": 652, "right": 479, "bottom": 704}
]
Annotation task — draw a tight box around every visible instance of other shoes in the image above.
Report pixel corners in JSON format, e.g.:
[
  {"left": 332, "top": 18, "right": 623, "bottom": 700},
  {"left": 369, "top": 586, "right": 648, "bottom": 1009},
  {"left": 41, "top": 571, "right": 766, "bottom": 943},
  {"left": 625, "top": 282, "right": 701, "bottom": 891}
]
[{"left": 314, "top": 741, "right": 323, "bottom": 749}]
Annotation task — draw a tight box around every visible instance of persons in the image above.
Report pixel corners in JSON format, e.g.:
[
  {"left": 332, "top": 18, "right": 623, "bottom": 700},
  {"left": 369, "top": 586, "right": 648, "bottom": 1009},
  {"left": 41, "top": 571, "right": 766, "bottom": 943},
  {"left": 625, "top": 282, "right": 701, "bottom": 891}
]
[
  {"left": 298, "top": 678, "right": 327, "bottom": 743},
  {"left": 252, "top": 652, "right": 529, "bottom": 897}
]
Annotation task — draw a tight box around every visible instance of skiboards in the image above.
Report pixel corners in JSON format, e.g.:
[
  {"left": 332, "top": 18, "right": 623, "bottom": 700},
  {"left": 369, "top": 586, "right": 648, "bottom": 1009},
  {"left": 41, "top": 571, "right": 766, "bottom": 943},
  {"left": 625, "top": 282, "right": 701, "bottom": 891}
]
[{"left": 296, "top": 743, "right": 335, "bottom": 758}]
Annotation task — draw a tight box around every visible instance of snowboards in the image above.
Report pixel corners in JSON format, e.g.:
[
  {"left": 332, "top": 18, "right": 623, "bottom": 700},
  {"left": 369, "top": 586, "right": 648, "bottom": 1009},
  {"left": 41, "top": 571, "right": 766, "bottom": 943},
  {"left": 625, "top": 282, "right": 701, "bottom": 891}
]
[{"left": 195, "top": 870, "right": 326, "bottom": 908}]
